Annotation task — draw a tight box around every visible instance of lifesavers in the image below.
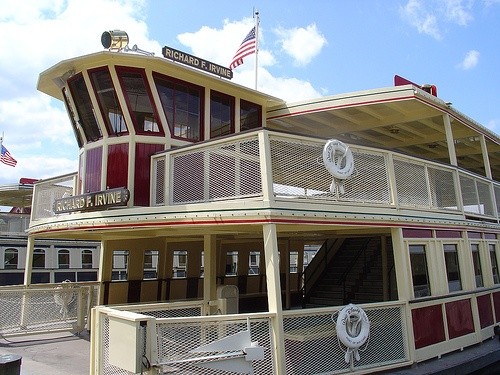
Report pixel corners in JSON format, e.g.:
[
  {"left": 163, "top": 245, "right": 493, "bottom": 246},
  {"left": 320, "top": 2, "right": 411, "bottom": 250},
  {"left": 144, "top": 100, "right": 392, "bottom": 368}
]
[
  {"left": 61, "top": 191, "right": 73, "bottom": 211},
  {"left": 336, "top": 303, "right": 371, "bottom": 350},
  {"left": 53, "top": 280, "right": 74, "bottom": 307},
  {"left": 322, "top": 138, "right": 355, "bottom": 180}
]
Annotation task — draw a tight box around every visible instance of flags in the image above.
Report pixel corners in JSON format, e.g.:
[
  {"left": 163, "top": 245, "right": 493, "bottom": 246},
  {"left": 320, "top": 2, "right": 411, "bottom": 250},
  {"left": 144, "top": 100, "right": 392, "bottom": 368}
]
[
  {"left": 0, "top": 145, "right": 17, "bottom": 167},
  {"left": 230, "top": 27, "right": 256, "bottom": 70}
]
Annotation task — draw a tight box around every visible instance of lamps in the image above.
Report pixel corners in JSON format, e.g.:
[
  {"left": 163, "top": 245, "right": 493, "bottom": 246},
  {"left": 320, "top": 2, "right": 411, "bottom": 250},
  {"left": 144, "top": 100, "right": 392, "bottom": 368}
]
[
  {"left": 428, "top": 142, "right": 436, "bottom": 149},
  {"left": 389, "top": 126, "right": 400, "bottom": 134}
]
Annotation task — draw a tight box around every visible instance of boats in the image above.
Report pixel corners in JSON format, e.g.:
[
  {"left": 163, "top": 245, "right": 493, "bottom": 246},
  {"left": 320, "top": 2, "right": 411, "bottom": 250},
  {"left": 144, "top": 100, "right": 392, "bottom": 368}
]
[{"left": 1, "top": 5, "right": 499, "bottom": 375}]
